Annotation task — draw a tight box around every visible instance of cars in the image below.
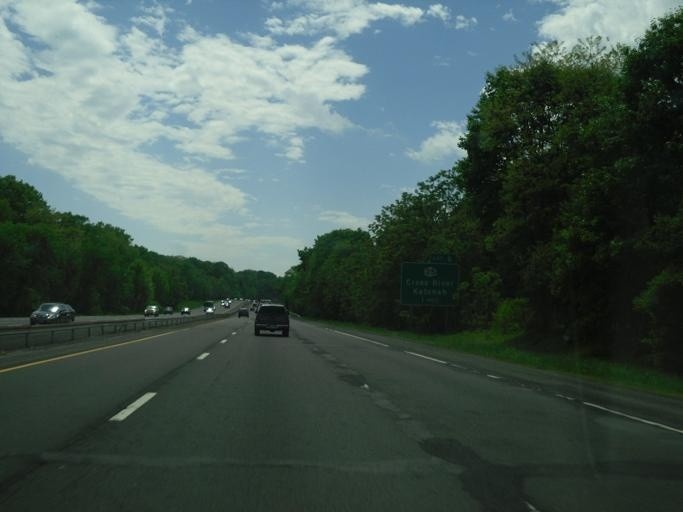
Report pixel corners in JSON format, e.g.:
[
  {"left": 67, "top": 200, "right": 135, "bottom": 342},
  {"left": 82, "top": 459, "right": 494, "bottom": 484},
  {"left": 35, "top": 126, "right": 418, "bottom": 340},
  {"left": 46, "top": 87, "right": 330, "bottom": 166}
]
[
  {"left": 29, "top": 300, "right": 75, "bottom": 326},
  {"left": 144, "top": 292, "right": 292, "bottom": 340}
]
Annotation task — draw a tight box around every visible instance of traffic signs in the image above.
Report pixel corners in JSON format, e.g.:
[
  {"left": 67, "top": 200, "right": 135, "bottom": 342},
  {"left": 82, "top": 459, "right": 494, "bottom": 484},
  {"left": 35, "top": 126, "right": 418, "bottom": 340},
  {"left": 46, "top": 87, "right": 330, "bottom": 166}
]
[{"left": 401, "top": 262, "right": 460, "bottom": 307}]
